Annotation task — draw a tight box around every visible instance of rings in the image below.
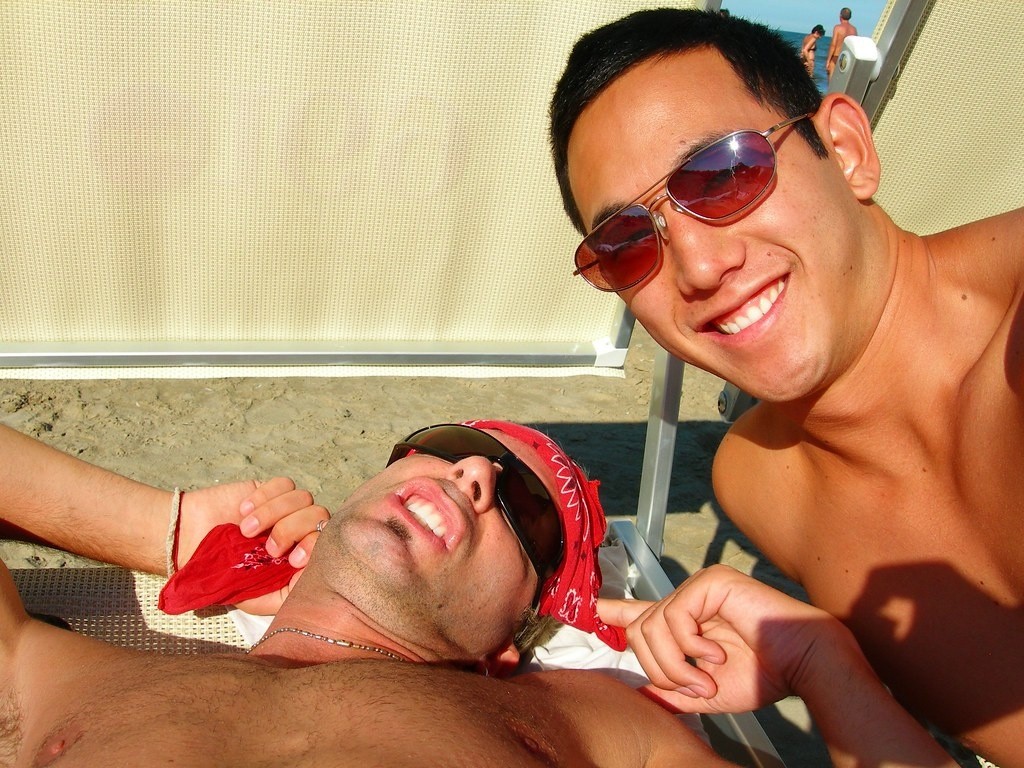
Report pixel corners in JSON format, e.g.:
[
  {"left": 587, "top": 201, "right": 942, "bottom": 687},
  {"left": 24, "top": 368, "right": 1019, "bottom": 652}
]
[{"left": 316, "top": 520, "right": 324, "bottom": 532}]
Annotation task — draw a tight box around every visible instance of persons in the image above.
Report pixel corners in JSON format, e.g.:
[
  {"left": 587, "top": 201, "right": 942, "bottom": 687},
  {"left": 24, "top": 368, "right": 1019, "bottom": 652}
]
[
  {"left": 801, "top": 8, "right": 857, "bottom": 94},
  {"left": 0, "top": 421, "right": 961, "bottom": 768},
  {"left": 549, "top": 7, "right": 1023, "bottom": 768}
]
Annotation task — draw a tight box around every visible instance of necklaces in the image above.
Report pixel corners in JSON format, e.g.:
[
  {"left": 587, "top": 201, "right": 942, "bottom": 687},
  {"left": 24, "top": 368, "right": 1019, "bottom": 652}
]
[{"left": 246, "top": 628, "right": 408, "bottom": 664}]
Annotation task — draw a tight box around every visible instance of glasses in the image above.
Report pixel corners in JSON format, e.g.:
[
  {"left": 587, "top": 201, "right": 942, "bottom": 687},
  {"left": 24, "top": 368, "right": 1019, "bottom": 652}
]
[
  {"left": 572, "top": 112, "right": 814, "bottom": 292},
  {"left": 386, "top": 423, "right": 565, "bottom": 612}
]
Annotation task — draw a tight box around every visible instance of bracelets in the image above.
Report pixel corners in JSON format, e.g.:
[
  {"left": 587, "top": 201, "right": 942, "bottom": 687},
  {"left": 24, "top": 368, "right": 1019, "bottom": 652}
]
[{"left": 166, "top": 486, "right": 184, "bottom": 579}]
